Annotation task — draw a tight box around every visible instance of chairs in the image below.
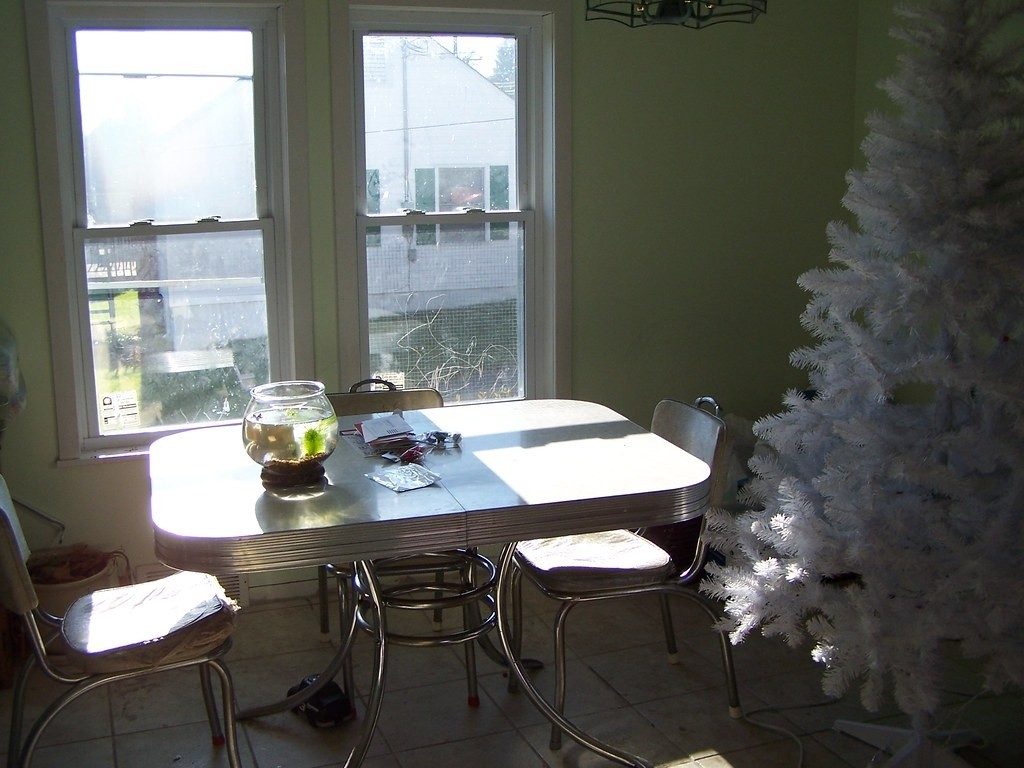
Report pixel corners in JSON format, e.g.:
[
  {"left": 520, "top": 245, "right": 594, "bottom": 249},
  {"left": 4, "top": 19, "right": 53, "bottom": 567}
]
[
  {"left": 316, "top": 378, "right": 481, "bottom": 717},
  {"left": 510, "top": 396, "right": 742, "bottom": 752},
  {"left": 0, "top": 472, "right": 243, "bottom": 768}
]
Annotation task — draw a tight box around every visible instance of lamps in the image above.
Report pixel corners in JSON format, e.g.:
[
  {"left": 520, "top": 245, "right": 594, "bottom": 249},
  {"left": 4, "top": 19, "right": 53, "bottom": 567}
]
[{"left": 585, "top": 0, "right": 768, "bottom": 30}]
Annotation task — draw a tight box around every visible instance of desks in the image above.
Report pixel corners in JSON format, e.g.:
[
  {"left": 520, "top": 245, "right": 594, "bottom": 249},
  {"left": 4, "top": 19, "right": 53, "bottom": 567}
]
[{"left": 144, "top": 397, "right": 712, "bottom": 768}]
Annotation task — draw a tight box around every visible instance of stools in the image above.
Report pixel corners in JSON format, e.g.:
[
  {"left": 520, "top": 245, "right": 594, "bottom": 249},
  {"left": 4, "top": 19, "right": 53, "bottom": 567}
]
[{"left": 26, "top": 542, "right": 136, "bottom": 593}]
